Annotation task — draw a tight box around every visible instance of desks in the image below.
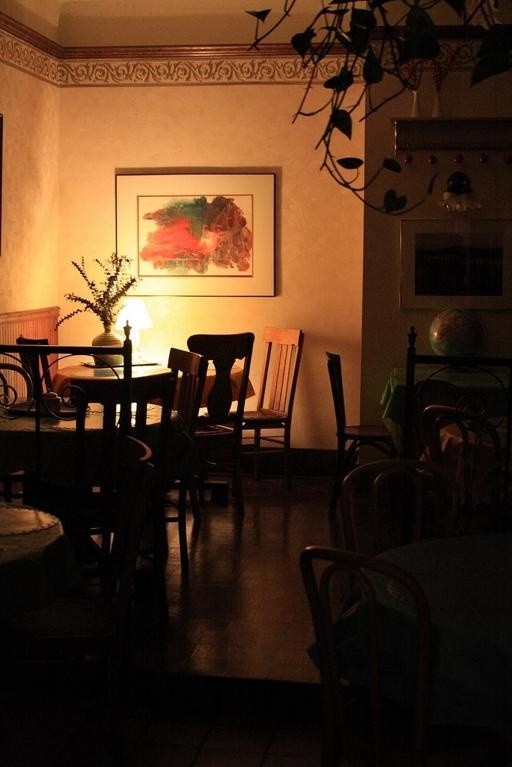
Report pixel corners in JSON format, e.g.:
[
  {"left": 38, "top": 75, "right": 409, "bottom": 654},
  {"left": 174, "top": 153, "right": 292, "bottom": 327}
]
[
  {"left": 434, "top": 421, "right": 511, "bottom": 517},
  {"left": 387, "top": 363, "right": 510, "bottom": 418}
]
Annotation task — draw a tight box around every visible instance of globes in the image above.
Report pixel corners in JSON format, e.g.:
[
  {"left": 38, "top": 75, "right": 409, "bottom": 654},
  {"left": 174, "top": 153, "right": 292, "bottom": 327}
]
[{"left": 429, "top": 308, "right": 487, "bottom": 373}]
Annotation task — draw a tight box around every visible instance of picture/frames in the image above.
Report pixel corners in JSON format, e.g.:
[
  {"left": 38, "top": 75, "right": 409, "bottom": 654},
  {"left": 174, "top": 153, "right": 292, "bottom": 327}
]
[
  {"left": 116, "top": 173, "right": 276, "bottom": 297},
  {"left": 399, "top": 219, "right": 512, "bottom": 311}
]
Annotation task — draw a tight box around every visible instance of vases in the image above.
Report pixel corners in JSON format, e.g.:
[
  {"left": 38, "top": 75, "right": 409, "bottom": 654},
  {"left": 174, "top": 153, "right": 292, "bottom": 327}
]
[
  {"left": 429, "top": 312, "right": 489, "bottom": 356},
  {"left": 409, "top": 90, "right": 422, "bottom": 118},
  {"left": 431, "top": 90, "right": 441, "bottom": 118}
]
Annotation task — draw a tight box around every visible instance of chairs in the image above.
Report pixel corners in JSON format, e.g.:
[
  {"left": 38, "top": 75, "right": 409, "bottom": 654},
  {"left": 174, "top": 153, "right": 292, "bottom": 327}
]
[
  {"left": 13, "top": 434, "right": 153, "bottom": 687},
  {"left": 295, "top": 542, "right": 435, "bottom": 765},
  {"left": 206, "top": 327, "right": 306, "bottom": 488},
  {"left": 418, "top": 403, "right": 502, "bottom": 536},
  {"left": 1, "top": 327, "right": 257, "bottom": 564},
  {"left": 324, "top": 349, "right": 394, "bottom": 490},
  {"left": 340, "top": 454, "right": 457, "bottom": 549}
]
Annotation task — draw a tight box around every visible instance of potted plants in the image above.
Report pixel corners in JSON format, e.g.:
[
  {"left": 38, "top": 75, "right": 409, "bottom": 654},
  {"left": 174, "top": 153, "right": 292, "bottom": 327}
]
[{"left": 55, "top": 253, "right": 143, "bottom": 369}]
